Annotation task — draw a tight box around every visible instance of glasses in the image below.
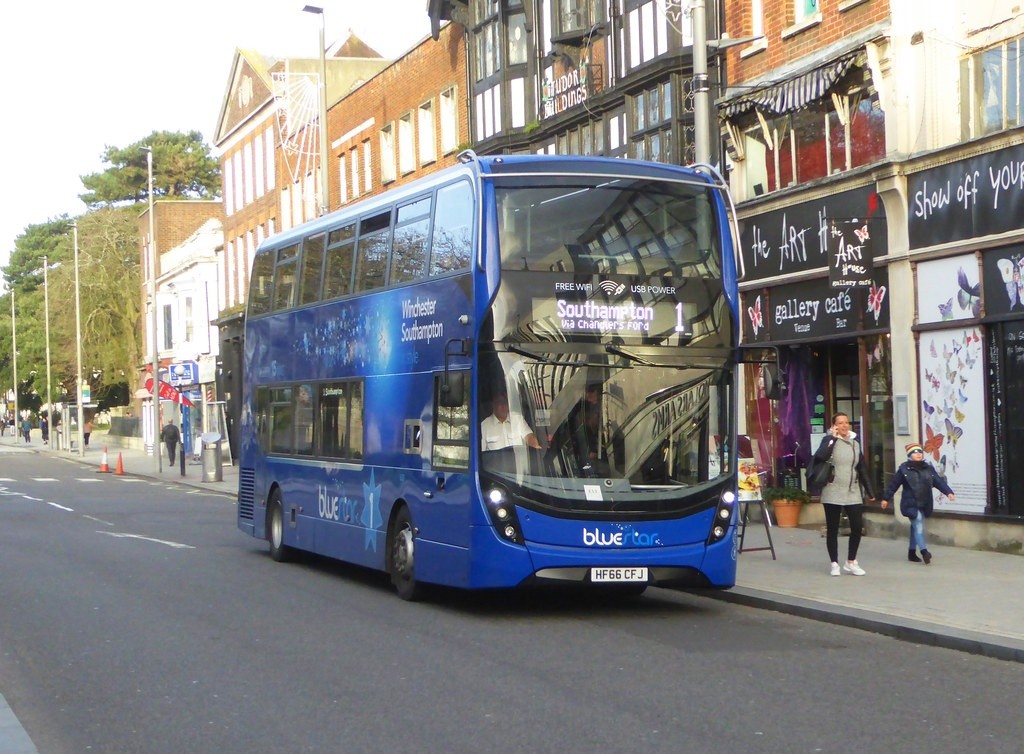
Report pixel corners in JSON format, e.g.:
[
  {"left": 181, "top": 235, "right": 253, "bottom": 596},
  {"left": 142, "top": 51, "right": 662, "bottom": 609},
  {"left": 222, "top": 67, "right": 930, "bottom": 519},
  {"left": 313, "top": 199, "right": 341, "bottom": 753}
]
[{"left": 911, "top": 450, "right": 923, "bottom": 453}]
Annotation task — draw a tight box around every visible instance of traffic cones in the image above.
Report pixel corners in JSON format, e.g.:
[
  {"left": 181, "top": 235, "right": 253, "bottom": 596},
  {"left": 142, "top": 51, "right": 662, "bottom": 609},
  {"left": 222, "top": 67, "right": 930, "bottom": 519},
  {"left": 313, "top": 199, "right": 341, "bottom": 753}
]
[
  {"left": 113, "top": 452, "right": 127, "bottom": 475},
  {"left": 96, "top": 446, "right": 113, "bottom": 473}
]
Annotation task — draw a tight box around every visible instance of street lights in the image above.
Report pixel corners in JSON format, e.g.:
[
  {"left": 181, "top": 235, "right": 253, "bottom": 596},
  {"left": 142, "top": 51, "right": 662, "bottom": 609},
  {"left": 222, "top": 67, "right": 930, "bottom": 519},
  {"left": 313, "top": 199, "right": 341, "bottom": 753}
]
[
  {"left": 302, "top": 4, "right": 330, "bottom": 215},
  {"left": 66, "top": 222, "right": 85, "bottom": 458},
  {"left": 138, "top": 145, "right": 161, "bottom": 472},
  {"left": 7, "top": 288, "right": 17, "bottom": 442},
  {"left": 38, "top": 255, "right": 53, "bottom": 450}
]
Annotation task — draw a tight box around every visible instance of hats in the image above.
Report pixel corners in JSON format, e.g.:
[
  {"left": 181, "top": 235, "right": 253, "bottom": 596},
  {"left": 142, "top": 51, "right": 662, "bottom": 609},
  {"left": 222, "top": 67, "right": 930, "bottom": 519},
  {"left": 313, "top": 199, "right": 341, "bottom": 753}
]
[{"left": 905, "top": 443, "right": 923, "bottom": 457}]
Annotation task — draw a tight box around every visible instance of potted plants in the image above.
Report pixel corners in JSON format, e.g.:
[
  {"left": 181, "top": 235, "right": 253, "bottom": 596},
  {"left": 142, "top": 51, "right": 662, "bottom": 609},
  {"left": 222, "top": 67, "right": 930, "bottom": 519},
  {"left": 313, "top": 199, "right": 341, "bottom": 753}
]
[{"left": 762, "top": 485, "right": 811, "bottom": 528}]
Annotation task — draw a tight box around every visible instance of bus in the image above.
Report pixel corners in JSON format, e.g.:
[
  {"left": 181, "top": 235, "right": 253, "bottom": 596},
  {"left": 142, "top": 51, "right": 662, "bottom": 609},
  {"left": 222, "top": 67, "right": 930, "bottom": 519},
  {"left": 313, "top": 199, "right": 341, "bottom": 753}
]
[{"left": 238, "top": 148, "right": 784, "bottom": 602}]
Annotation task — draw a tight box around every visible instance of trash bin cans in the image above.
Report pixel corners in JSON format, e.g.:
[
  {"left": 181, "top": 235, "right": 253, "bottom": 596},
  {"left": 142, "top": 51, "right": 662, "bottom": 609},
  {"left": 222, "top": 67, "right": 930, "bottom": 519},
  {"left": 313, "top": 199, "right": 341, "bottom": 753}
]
[
  {"left": 777, "top": 453, "right": 801, "bottom": 489},
  {"left": 201, "top": 432, "right": 222, "bottom": 482}
]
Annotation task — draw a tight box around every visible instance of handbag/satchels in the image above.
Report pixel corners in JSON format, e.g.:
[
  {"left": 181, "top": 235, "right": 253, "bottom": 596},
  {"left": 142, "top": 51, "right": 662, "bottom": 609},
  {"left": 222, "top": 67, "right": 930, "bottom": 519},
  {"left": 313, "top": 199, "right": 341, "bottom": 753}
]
[
  {"left": 161, "top": 442, "right": 167, "bottom": 455},
  {"left": 806, "top": 459, "right": 835, "bottom": 488}
]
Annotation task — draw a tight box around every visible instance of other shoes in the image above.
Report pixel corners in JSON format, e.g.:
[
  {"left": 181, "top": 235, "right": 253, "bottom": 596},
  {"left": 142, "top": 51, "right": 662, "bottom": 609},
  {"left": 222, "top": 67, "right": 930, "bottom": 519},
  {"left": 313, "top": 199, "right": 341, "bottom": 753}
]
[
  {"left": 923, "top": 552, "right": 931, "bottom": 564},
  {"left": 909, "top": 553, "right": 921, "bottom": 562}
]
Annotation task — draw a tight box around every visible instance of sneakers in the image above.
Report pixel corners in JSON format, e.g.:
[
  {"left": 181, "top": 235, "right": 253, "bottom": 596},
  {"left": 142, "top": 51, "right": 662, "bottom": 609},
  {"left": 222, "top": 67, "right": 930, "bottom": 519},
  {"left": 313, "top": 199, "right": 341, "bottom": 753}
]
[
  {"left": 831, "top": 562, "right": 841, "bottom": 576},
  {"left": 843, "top": 559, "right": 865, "bottom": 576}
]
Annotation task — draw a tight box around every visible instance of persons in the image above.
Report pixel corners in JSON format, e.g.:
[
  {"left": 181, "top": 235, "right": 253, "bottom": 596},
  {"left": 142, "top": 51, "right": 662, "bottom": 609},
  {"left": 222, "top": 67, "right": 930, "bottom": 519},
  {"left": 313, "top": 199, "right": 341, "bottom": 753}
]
[
  {"left": 0, "top": 414, "right": 48, "bottom": 445},
  {"left": 811, "top": 412, "right": 875, "bottom": 576},
  {"left": 881, "top": 443, "right": 955, "bottom": 565},
  {"left": 481, "top": 396, "right": 542, "bottom": 451},
  {"left": 84, "top": 420, "right": 92, "bottom": 449},
  {"left": 161, "top": 418, "right": 182, "bottom": 467}
]
